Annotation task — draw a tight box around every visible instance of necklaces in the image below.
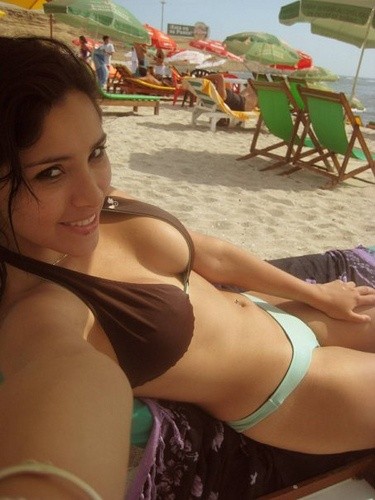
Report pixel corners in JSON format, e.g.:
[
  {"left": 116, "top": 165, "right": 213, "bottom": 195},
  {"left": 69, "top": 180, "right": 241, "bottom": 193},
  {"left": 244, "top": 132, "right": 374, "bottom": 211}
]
[{"left": 46, "top": 253, "right": 69, "bottom": 267}]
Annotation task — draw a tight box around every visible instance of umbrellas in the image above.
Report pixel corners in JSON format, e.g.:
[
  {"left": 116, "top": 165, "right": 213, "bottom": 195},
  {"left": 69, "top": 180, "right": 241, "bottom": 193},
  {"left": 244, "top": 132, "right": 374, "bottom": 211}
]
[{"left": 42, "top": 1, "right": 375, "bottom": 125}]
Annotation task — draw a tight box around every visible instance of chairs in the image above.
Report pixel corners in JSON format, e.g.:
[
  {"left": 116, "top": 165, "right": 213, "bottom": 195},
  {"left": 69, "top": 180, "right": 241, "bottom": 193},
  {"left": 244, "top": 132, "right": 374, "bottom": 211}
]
[
  {"left": 90, "top": 62, "right": 209, "bottom": 115},
  {"left": 130, "top": 244, "right": 375, "bottom": 500},
  {"left": 235, "top": 71, "right": 375, "bottom": 190},
  {"left": 183, "top": 76, "right": 261, "bottom": 132}
]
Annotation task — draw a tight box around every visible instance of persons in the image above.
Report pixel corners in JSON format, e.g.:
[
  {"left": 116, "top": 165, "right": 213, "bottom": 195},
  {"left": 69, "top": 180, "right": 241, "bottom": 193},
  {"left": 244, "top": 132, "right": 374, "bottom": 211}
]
[
  {"left": 202, "top": 73, "right": 258, "bottom": 110},
  {"left": 0, "top": 33, "right": 375, "bottom": 500},
  {"left": 78, "top": 34, "right": 166, "bottom": 88}
]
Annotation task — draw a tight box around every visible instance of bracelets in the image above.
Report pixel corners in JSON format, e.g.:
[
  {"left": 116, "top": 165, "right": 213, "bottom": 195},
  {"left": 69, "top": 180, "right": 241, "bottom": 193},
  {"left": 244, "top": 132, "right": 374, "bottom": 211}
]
[{"left": 0, "top": 459, "right": 103, "bottom": 500}]
